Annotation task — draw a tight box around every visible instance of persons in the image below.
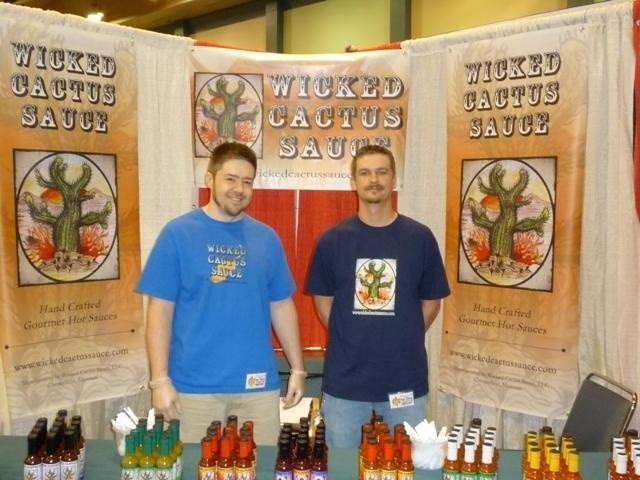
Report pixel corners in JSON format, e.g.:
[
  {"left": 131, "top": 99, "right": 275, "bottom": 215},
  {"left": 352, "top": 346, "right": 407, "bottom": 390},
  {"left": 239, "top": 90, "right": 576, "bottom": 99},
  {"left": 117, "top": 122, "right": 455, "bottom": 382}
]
[
  {"left": 303, "top": 144, "right": 453, "bottom": 451},
  {"left": 132, "top": 141, "right": 311, "bottom": 450}
]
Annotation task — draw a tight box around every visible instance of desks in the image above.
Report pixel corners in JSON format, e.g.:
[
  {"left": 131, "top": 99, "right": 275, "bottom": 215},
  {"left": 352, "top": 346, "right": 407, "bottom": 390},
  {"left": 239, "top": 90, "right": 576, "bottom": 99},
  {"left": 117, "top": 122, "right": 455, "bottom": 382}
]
[{"left": 1, "top": 428, "right": 638, "bottom": 478}]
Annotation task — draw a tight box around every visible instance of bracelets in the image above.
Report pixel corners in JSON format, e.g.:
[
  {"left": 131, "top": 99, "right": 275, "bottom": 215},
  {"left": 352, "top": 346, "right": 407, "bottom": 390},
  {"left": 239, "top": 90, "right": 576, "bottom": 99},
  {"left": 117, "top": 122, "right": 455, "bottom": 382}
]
[
  {"left": 288, "top": 367, "right": 309, "bottom": 379},
  {"left": 146, "top": 375, "right": 173, "bottom": 390}
]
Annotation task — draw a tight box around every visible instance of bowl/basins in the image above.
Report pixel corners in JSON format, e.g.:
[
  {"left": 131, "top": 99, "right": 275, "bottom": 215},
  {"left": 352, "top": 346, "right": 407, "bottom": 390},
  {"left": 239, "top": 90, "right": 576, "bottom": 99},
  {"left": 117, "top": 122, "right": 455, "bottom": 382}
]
[
  {"left": 405, "top": 431, "right": 448, "bottom": 470},
  {"left": 112, "top": 418, "right": 171, "bottom": 458}
]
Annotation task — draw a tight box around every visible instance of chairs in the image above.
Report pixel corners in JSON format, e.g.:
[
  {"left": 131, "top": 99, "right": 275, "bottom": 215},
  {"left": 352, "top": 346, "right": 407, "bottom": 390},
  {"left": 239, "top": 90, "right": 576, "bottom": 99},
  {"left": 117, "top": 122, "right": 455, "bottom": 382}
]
[{"left": 556, "top": 369, "right": 639, "bottom": 453}]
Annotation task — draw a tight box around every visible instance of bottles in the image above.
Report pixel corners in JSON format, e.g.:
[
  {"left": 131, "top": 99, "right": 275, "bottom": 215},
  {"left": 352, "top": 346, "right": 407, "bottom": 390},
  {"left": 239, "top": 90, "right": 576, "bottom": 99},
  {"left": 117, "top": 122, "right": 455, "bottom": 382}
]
[
  {"left": 197, "top": 414, "right": 259, "bottom": 480},
  {"left": 443, "top": 418, "right": 500, "bottom": 480},
  {"left": 274, "top": 415, "right": 329, "bottom": 480},
  {"left": 522, "top": 426, "right": 583, "bottom": 480},
  {"left": 121, "top": 413, "right": 184, "bottom": 480},
  {"left": 607, "top": 428, "right": 639, "bottom": 480},
  {"left": 357, "top": 416, "right": 415, "bottom": 480},
  {"left": 21, "top": 409, "right": 88, "bottom": 480}
]
